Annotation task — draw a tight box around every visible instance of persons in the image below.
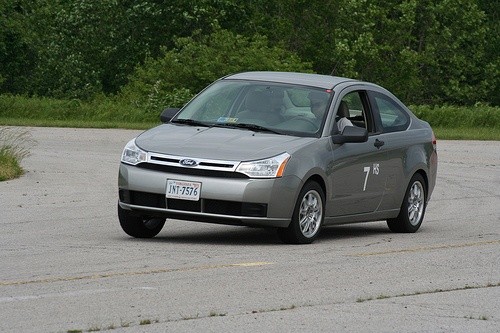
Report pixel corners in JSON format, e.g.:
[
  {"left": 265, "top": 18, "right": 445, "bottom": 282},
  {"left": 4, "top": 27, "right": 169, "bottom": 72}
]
[{"left": 305, "top": 90, "right": 354, "bottom": 138}]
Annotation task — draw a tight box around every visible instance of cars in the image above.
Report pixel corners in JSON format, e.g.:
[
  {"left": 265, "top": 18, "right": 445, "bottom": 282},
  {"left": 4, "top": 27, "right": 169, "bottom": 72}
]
[{"left": 117, "top": 71, "right": 438, "bottom": 244}]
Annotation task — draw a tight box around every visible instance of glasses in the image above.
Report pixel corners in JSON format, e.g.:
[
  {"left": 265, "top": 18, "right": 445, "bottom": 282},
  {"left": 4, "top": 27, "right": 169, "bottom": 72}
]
[{"left": 310, "top": 101, "right": 322, "bottom": 108}]
